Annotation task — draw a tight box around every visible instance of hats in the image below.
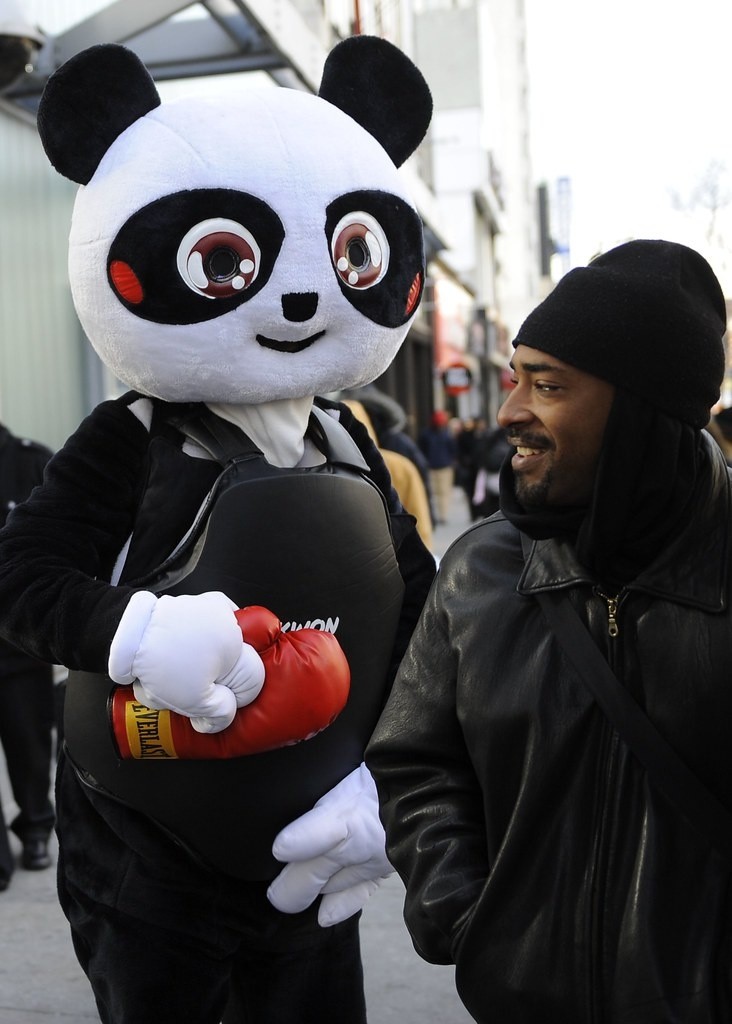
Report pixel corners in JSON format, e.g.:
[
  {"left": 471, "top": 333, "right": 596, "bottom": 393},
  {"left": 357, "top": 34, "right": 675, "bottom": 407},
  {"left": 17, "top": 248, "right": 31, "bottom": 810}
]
[{"left": 512, "top": 238, "right": 727, "bottom": 428}]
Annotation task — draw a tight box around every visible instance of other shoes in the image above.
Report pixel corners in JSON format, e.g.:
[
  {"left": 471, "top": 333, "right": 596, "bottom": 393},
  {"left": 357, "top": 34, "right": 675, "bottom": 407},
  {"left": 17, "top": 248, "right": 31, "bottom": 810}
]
[{"left": 20, "top": 839, "right": 52, "bottom": 870}]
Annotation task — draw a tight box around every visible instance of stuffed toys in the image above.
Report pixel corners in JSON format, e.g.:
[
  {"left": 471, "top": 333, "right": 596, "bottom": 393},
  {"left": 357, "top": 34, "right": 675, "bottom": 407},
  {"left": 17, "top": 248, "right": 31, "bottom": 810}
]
[{"left": 1, "top": 35, "right": 436, "bottom": 1024}]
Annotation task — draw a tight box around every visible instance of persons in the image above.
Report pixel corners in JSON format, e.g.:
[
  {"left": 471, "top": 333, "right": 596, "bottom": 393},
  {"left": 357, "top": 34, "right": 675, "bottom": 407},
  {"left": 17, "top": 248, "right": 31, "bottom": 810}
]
[
  {"left": 1, "top": 424, "right": 54, "bottom": 870},
  {"left": 367, "top": 241, "right": 732, "bottom": 1024},
  {"left": 343, "top": 385, "right": 515, "bottom": 545}
]
